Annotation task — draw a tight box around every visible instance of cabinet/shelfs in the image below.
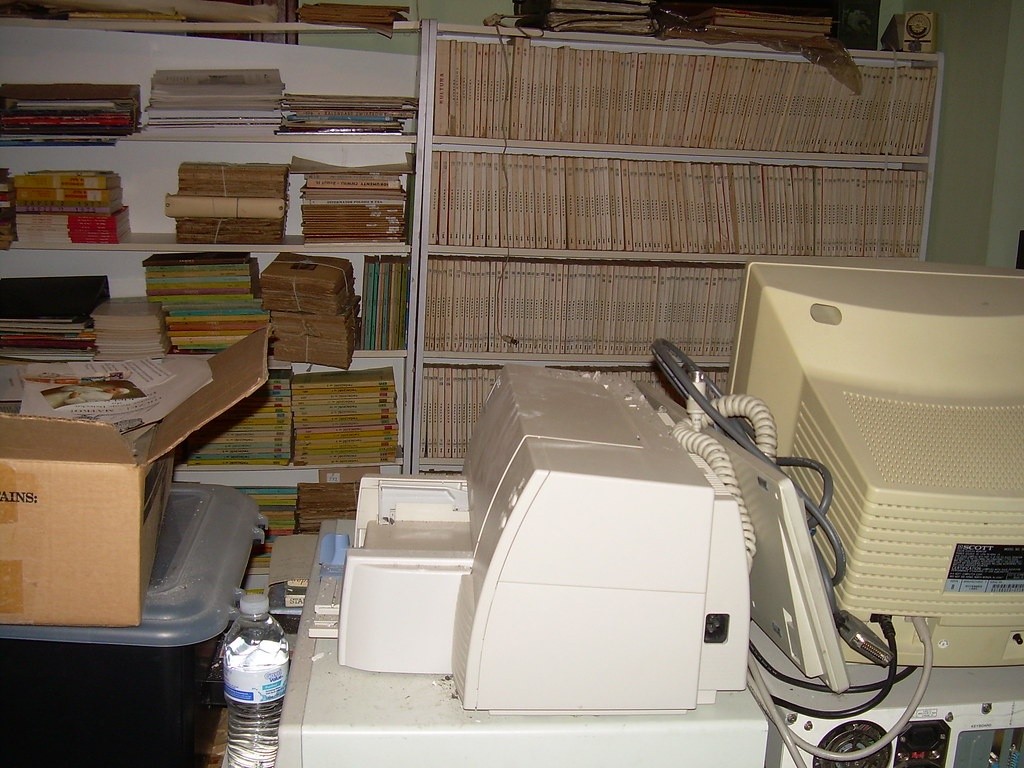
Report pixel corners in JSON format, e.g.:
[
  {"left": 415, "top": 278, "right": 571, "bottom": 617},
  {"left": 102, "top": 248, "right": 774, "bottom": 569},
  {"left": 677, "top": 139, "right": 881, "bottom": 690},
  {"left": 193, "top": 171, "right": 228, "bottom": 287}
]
[
  {"left": 273, "top": 519, "right": 771, "bottom": 766},
  {"left": 0, "top": 12, "right": 943, "bottom": 476}
]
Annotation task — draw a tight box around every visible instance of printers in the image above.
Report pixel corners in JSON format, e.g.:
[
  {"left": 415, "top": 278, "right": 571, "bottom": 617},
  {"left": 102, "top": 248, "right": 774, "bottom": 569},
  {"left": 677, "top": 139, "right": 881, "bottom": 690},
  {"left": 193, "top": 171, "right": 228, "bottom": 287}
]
[{"left": 307, "top": 365, "right": 749, "bottom": 713}]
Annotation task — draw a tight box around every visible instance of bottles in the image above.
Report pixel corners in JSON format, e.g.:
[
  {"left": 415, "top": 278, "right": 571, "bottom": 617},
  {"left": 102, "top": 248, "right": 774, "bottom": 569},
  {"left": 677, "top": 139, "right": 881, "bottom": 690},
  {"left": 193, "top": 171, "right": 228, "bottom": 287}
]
[{"left": 221, "top": 594, "right": 290, "bottom": 768}]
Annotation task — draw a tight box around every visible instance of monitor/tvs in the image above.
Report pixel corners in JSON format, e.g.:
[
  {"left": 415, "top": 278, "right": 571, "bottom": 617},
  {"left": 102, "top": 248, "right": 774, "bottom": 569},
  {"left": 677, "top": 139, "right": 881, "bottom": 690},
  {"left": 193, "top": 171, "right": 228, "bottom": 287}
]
[{"left": 727, "top": 257, "right": 1022, "bottom": 668}]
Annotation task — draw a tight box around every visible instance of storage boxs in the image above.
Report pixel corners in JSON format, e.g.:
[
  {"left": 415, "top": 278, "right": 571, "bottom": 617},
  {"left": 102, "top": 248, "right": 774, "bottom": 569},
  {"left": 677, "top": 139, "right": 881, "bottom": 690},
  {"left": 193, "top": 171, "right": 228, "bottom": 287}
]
[
  {"left": 0, "top": 321, "right": 275, "bottom": 626},
  {"left": 0, "top": 482, "right": 269, "bottom": 768}
]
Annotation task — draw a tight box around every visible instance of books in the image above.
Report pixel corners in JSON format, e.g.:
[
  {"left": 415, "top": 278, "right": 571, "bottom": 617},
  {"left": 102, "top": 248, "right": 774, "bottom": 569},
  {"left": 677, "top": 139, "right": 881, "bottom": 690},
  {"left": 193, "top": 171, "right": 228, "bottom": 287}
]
[
  {"left": 0, "top": 151, "right": 416, "bottom": 251},
  {"left": 0, "top": 0, "right": 412, "bottom": 38},
  {"left": 0, "top": 69, "right": 419, "bottom": 146},
  {"left": 419, "top": 363, "right": 729, "bottom": 460},
  {"left": 0, "top": 251, "right": 411, "bottom": 370},
  {"left": 1, "top": 366, "right": 405, "bottom": 614},
  {"left": 513, "top": 0, "right": 832, "bottom": 50},
  {"left": 428, "top": 152, "right": 927, "bottom": 258},
  {"left": 434, "top": 33, "right": 937, "bottom": 156},
  {"left": 422, "top": 255, "right": 744, "bottom": 356}
]
